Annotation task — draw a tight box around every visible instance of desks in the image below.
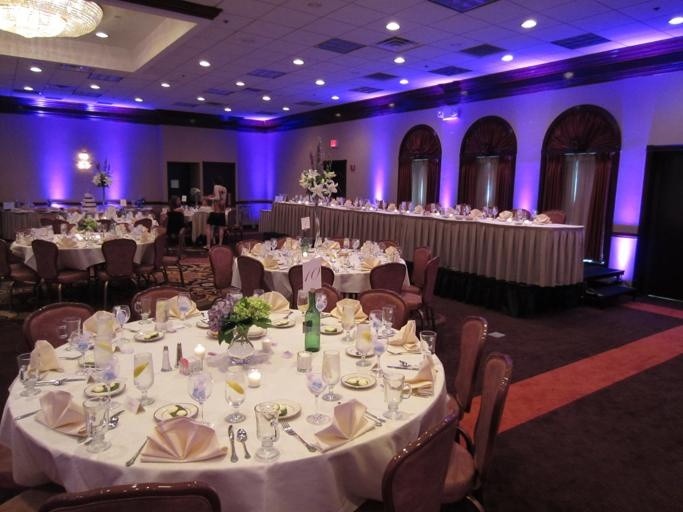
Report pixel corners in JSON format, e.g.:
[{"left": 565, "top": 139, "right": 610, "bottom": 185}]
[{"left": 271, "top": 200, "right": 588, "bottom": 288}]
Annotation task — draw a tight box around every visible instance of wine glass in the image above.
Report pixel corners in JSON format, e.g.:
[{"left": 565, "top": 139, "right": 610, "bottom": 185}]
[
  {"left": 295, "top": 195, "right": 312, "bottom": 204},
  {"left": 431, "top": 203, "right": 537, "bottom": 225},
  {"left": 16, "top": 291, "right": 215, "bottom": 453},
  {"left": 241, "top": 238, "right": 400, "bottom": 272},
  {"left": 339, "top": 196, "right": 413, "bottom": 214},
  {"left": 16, "top": 223, "right": 148, "bottom": 248},
  {"left": 225, "top": 288, "right": 438, "bottom": 462}
]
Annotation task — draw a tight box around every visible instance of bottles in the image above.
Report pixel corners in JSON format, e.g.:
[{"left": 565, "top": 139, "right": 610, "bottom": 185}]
[{"left": 302, "top": 231, "right": 309, "bottom": 258}]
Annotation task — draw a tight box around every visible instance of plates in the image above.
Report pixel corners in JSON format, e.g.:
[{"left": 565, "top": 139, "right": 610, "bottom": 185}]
[
  {"left": 78, "top": 318, "right": 218, "bottom": 421},
  {"left": 246, "top": 318, "right": 376, "bottom": 420}
]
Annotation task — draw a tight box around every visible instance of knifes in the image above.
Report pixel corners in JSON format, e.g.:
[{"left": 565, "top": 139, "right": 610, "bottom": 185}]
[{"left": 126, "top": 438, "right": 148, "bottom": 466}]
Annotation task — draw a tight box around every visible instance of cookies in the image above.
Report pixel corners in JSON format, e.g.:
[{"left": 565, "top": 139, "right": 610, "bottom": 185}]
[
  {"left": 346, "top": 378, "right": 365, "bottom": 386},
  {"left": 324, "top": 327, "right": 336, "bottom": 333},
  {"left": 168, "top": 404, "right": 188, "bottom": 417},
  {"left": 143, "top": 331, "right": 160, "bottom": 339},
  {"left": 274, "top": 404, "right": 287, "bottom": 417}
]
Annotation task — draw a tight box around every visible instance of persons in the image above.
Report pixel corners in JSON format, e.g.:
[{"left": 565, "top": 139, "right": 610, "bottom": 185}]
[
  {"left": 202, "top": 175, "right": 228, "bottom": 250},
  {"left": 166, "top": 195, "right": 184, "bottom": 234}
]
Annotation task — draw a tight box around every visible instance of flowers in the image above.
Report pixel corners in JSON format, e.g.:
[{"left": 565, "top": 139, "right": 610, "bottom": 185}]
[
  {"left": 94, "top": 160, "right": 112, "bottom": 189},
  {"left": 299, "top": 140, "right": 340, "bottom": 203}
]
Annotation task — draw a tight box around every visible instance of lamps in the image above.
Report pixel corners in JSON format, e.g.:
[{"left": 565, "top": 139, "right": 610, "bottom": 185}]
[{"left": 0, "top": 0, "right": 104, "bottom": 39}]
